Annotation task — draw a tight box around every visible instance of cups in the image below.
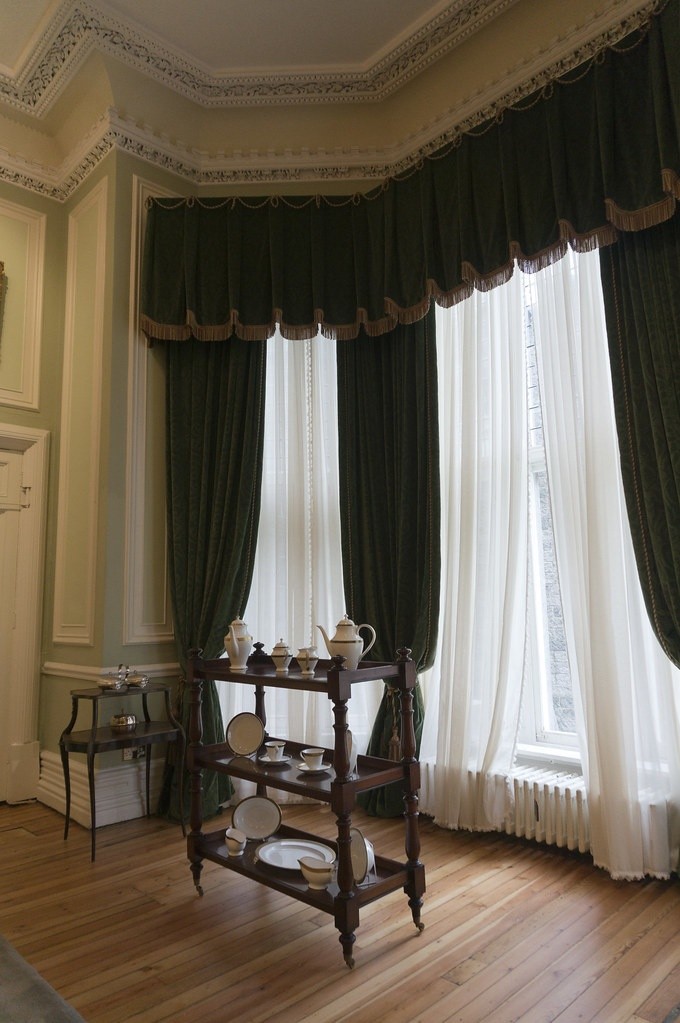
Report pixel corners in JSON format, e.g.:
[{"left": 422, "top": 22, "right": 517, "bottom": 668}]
[
  {"left": 297, "top": 856, "right": 334, "bottom": 890},
  {"left": 225, "top": 827, "right": 246, "bottom": 857},
  {"left": 300, "top": 748, "right": 325, "bottom": 768},
  {"left": 264, "top": 741, "right": 286, "bottom": 761}
]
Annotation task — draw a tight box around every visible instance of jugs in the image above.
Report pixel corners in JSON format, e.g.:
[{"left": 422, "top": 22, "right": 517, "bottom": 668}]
[
  {"left": 271, "top": 638, "right": 293, "bottom": 672},
  {"left": 224, "top": 616, "right": 253, "bottom": 669},
  {"left": 316, "top": 614, "right": 376, "bottom": 670},
  {"left": 296, "top": 647, "right": 319, "bottom": 675}
]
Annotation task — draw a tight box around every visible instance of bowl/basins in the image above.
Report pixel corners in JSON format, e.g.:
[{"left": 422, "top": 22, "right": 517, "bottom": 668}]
[{"left": 350, "top": 827, "right": 375, "bottom": 884}]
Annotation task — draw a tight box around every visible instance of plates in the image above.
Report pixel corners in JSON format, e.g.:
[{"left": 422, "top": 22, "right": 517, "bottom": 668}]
[
  {"left": 258, "top": 754, "right": 292, "bottom": 766},
  {"left": 226, "top": 712, "right": 264, "bottom": 756},
  {"left": 255, "top": 838, "right": 336, "bottom": 870},
  {"left": 296, "top": 762, "right": 332, "bottom": 775},
  {"left": 345, "top": 730, "right": 358, "bottom": 781},
  {"left": 232, "top": 795, "right": 282, "bottom": 840}
]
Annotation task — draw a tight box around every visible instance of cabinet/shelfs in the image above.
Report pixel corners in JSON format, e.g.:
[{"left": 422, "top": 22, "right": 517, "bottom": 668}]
[{"left": 173, "top": 648, "right": 429, "bottom": 975}]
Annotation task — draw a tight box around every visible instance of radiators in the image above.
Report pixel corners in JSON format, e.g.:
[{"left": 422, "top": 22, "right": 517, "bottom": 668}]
[{"left": 415, "top": 756, "right": 672, "bottom": 857}]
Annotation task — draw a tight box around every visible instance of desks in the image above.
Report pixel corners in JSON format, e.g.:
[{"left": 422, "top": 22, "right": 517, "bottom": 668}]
[{"left": 58, "top": 681, "right": 188, "bottom": 861}]
